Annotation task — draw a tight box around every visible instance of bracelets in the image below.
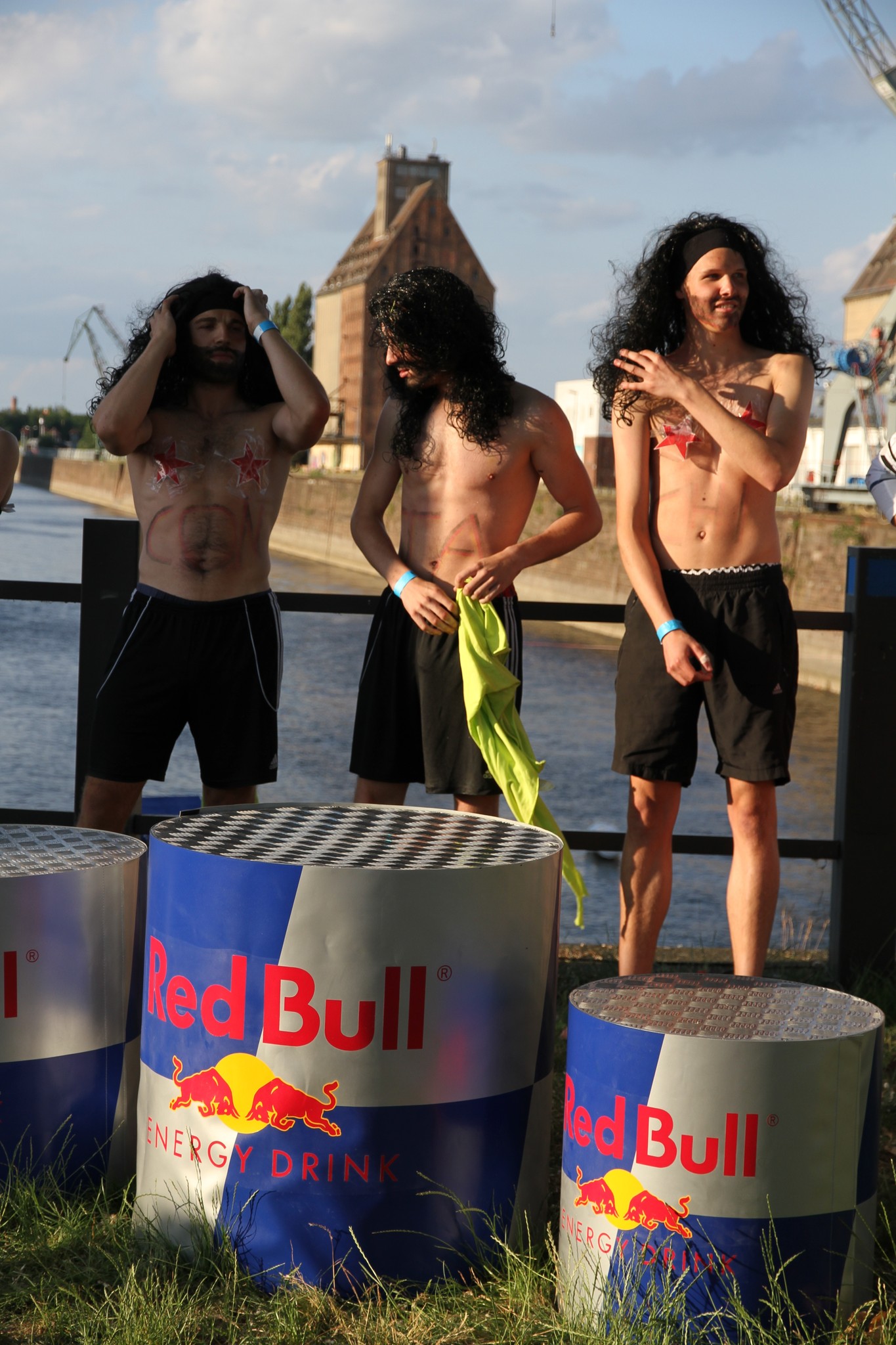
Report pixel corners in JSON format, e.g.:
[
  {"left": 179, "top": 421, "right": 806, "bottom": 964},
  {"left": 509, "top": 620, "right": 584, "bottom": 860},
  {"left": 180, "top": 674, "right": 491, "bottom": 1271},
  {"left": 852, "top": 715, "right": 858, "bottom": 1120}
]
[
  {"left": 253, "top": 320, "right": 280, "bottom": 344},
  {"left": 393, "top": 570, "right": 418, "bottom": 597},
  {"left": 656, "top": 620, "right": 685, "bottom": 644}
]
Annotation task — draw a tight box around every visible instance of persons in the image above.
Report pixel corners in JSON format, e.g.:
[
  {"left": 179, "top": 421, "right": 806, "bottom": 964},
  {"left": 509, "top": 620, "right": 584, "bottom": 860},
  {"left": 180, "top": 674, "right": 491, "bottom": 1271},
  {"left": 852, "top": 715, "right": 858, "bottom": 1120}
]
[
  {"left": 585, "top": 210, "right": 831, "bottom": 981},
  {"left": 349, "top": 266, "right": 603, "bottom": 816},
  {"left": 78, "top": 273, "right": 332, "bottom": 830},
  {"left": 864, "top": 431, "right": 896, "bottom": 530}
]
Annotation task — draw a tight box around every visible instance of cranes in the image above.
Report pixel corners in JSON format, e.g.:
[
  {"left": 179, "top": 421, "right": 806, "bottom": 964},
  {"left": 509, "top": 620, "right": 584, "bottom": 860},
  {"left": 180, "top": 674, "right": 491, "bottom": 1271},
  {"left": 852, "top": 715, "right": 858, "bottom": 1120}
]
[{"left": 62, "top": 306, "right": 129, "bottom": 382}]
